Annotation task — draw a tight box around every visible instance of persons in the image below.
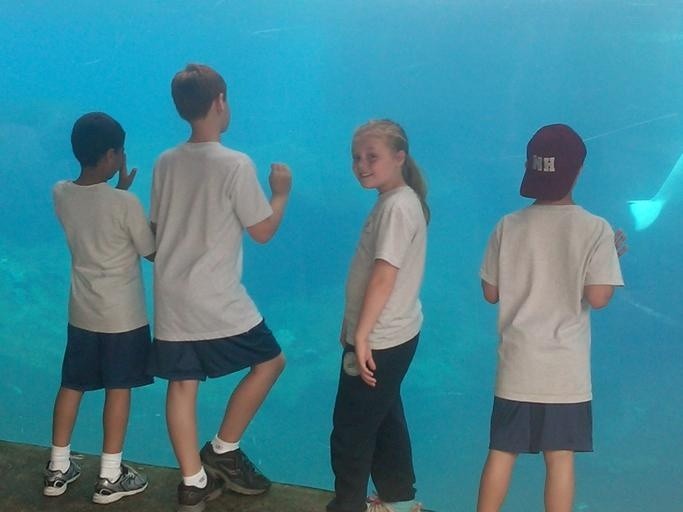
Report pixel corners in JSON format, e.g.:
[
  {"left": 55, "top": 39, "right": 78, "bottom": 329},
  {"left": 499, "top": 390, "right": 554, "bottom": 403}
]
[
  {"left": 477, "top": 125, "right": 629, "bottom": 512},
  {"left": 44, "top": 111, "right": 155, "bottom": 503},
  {"left": 145, "top": 63, "right": 291, "bottom": 512},
  {"left": 324, "top": 119, "right": 430, "bottom": 512}
]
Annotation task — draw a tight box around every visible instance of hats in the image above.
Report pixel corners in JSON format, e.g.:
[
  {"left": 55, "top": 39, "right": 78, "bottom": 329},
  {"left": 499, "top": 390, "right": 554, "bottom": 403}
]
[{"left": 520, "top": 124, "right": 587, "bottom": 200}]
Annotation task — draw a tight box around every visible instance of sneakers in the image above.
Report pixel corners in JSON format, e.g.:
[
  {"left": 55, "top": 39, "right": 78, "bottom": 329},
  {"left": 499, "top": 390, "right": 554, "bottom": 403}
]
[
  {"left": 92, "top": 463, "right": 150, "bottom": 504},
  {"left": 176, "top": 441, "right": 271, "bottom": 512},
  {"left": 42, "top": 458, "right": 81, "bottom": 496},
  {"left": 364, "top": 490, "right": 422, "bottom": 512}
]
